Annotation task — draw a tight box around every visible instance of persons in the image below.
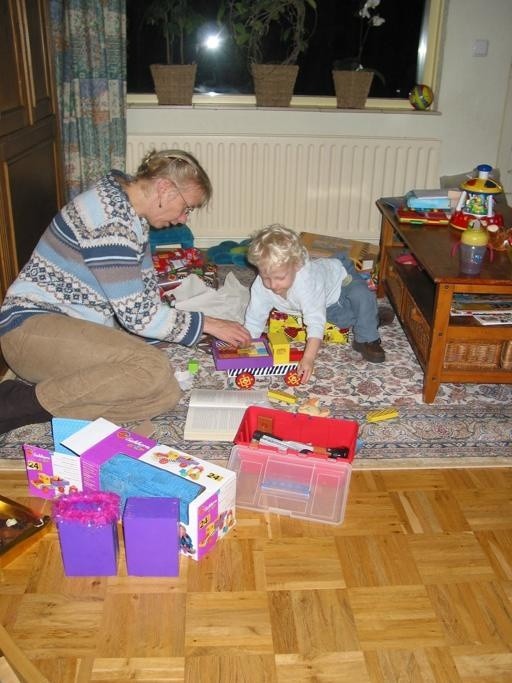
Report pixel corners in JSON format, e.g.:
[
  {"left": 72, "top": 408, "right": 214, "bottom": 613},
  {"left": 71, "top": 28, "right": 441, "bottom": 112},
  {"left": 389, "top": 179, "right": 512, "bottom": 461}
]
[
  {"left": 1, "top": 147, "right": 252, "bottom": 438},
  {"left": 242, "top": 224, "right": 399, "bottom": 390}
]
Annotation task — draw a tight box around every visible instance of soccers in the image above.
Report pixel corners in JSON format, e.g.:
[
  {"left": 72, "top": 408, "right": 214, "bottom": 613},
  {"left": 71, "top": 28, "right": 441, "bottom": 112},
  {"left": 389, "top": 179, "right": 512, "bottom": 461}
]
[{"left": 410, "top": 85, "right": 432, "bottom": 110}]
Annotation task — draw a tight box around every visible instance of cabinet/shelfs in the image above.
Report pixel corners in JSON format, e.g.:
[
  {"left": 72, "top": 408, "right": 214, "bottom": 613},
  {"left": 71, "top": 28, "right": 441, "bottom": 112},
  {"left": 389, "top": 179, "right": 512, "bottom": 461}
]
[
  {"left": 0, "top": 0, "right": 69, "bottom": 383},
  {"left": 374, "top": 189, "right": 512, "bottom": 402}
]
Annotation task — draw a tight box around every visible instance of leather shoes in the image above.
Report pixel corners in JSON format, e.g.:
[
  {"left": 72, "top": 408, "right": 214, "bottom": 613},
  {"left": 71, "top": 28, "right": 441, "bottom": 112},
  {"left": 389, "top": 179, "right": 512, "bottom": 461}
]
[
  {"left": 377, "top": 307, "right": 395, "bottom": 326},
  {"left": 352, "top": 337, "right": 385, "bottom": 363}
]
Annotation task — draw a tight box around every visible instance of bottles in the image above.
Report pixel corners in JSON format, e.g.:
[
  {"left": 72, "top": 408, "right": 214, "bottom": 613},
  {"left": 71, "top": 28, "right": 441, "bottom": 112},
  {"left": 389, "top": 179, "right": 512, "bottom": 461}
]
[{"left": 456, "top": 216, "right": 488, "bottom": 277}]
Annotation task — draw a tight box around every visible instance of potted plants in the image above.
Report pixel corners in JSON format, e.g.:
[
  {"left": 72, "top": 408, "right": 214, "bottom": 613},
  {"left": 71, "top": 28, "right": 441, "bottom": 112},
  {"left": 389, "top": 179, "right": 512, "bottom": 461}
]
[{"left": 141, "top": 0, "right": 318, "bottom": 107}]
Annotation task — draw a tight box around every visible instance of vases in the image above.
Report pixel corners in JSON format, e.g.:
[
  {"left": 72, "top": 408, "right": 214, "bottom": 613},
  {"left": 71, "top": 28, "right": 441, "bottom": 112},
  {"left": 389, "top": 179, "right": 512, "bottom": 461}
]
[{"left": 330, "top": 67, "right": 374, "bottom": 109}]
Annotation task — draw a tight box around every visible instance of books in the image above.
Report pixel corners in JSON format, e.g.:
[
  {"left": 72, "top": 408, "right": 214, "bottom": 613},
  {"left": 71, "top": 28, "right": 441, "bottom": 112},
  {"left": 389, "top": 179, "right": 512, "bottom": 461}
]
[{"left": 184, "top": 389, "right": 267, "bottom": 442}]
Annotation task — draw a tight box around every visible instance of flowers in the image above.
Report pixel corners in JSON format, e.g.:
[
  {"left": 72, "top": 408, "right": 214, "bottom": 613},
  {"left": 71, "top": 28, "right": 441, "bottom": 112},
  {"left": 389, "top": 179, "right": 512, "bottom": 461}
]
[{"left": 333, "top": 0, "right": 387, "bottom": 82}]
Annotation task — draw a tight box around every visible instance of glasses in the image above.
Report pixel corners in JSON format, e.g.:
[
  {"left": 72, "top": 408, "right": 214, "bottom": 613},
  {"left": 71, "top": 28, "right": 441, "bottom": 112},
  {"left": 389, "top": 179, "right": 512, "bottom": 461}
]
[{"left": 169, "top": 179, "right": 194, "bottom": 216}]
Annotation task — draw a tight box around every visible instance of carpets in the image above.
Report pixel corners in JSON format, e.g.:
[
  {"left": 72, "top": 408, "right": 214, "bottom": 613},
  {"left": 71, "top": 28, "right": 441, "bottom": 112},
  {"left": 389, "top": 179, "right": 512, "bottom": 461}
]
[{"left": 0, "top": 262, "right": 512, "bottom": 468}]
